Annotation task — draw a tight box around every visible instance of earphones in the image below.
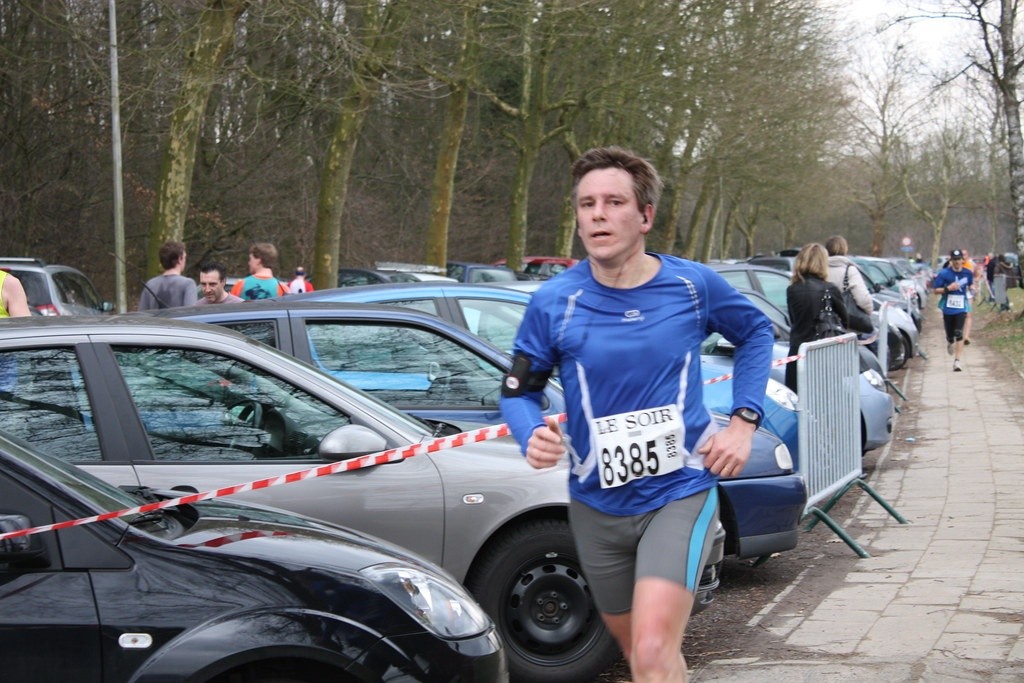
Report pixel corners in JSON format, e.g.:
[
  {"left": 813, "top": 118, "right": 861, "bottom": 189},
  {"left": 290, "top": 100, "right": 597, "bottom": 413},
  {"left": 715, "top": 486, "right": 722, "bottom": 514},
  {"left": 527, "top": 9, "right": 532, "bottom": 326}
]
[{"left": 643, "top": 216, "right": 648, "bottom": 223}]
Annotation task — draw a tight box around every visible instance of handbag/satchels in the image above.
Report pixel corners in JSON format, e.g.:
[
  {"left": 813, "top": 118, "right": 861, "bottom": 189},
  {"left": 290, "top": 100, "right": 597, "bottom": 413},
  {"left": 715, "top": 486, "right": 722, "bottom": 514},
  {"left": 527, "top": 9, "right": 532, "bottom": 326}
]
[
  {"left": 813, "top": 281, "right": 846, "bottom": 340},
  {"left": 840, "top": 264, "right": 874, "bottom": 334}
]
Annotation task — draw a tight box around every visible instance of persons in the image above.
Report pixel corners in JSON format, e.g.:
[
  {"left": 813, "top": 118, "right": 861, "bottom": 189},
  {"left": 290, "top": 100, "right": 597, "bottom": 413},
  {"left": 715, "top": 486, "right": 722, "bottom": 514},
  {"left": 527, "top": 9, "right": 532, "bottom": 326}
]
[
  {"left": 287, "top": 266, "right": 313, "bottom": 296},
  {"left": 787, "top": 242, "right": 879, "bottom": 479},
  {"left": 136, "top": 242, "right": 199, "bottom": 310},
  {"left": 227, "top": 242, "right": 291, "bottom": 301},
  {"left": 960, "top": 247, "right": 980, "bottom": 345},
  {"left": 195, "top": 261, "right": 251, "bottom": 305},
  {"left": 0, "top": 270, "right": 32, "bottom": 392},
  {"left": 983, "top": 254, "right": 1017, "bottom": 310},
  {"left": 500, "top": 146, "right": 774, "bottom": 683},
  {"left": 825, "top": 235, "right": 879, "bottom": 358},
  {"left": 933, "top": 248, "right": 976, "bottom": 373}
]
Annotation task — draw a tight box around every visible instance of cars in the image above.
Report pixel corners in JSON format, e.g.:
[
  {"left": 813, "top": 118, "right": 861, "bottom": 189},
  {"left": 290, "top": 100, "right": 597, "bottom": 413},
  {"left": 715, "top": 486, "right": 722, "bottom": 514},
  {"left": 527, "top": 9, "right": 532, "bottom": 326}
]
[
  {"left": 249, "top": 246, "right": 937, "bottom": 578},
  {"left": 0, "top": 428, "right": 515, "bottom": 682},
  {"left": 90, "top": 295, "right": 810, "bottom": 683},
  {"left": 938, "top": 252, "right": 1020, "bottom": 287}
]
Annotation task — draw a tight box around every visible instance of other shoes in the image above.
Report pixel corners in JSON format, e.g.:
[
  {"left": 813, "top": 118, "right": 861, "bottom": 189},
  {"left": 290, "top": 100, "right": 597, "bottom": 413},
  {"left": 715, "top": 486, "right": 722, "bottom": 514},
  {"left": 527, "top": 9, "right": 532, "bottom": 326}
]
[
  {"left": 953, "top": 364, "right": 962, "bottom": 371},
  {"left": 963, "top": 339, "right": 970, "bottom": 345},
  {"left": 947, "top": 342, "right": 955, "bottom": 354}
]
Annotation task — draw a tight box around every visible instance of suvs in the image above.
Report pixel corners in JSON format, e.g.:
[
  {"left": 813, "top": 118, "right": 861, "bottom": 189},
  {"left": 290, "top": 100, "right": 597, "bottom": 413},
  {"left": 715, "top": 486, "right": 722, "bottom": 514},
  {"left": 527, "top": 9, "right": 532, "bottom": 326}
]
[
  {"left": 0, "top": 257, "right": 117, "bottom": 320},
  {"left": 0, "top": 300, "right": 726, "bottom": 683}
]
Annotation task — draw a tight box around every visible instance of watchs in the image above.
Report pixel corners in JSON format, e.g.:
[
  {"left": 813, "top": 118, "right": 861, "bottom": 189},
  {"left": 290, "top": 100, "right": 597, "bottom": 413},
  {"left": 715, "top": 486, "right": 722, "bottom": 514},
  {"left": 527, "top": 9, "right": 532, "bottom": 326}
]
[
  {"left": 731, "top": 408, "right": 761, "bottom": 432},
  {"left": 943, "top": 286, "right": 949, "bottom": 293}
]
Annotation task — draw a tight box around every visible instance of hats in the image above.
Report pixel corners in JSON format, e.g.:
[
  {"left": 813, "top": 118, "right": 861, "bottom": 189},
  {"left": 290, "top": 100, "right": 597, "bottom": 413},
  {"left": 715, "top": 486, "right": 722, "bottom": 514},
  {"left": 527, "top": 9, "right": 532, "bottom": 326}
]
[{"left": 951, "top": 250, "right": 963, "bottom": 260}]
